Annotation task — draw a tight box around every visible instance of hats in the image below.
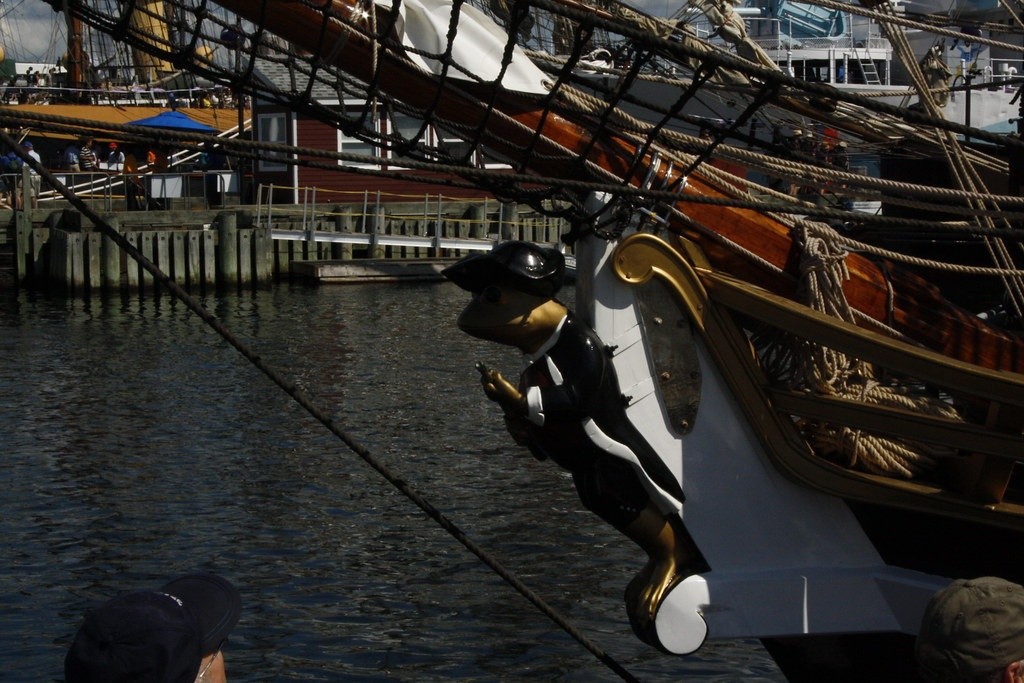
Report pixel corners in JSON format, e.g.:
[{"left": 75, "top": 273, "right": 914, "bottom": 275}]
[
  {"left": 63, "top": 572, "right": 243, "bottom": 683},
  {"left": 23, "top": 141, "right": 33, "bottom": 147},
  {"left": 109, "top": 143, "right": 117, "bottom": 147}
]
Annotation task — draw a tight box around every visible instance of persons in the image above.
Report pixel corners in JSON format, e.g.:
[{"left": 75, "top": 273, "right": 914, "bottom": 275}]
[
  {"left": 64, "top": 572, "right": 240, "bottom": 683},
  {"left": 62, "top": 140, "right": 81, "bottom": 174},
  {"left": 23, "top": 67, "right": 56, "bottom": 87},
  {"left": 202, "top": 86, "right": 249, "bottom": 110},
  {"left": 124, "top": 150, "right": 139, "bottom": 211},
  {"left": 440, "top": 241, "right": 685, "bottom": 623},
  {"left": 917, "top": 576, "right": 1024, "bottom": 683},
  {"left": 79, "top": 136, "right": 100, "bottom": 172},
  {"left": 147, "top": 143, "right": 157, "bottom": 169},
  {"left": 0, "top": 142, "right": 41, "bottom": 209},
  {"left": 107, "top": 142, "right": 125, "bottom": 173}
]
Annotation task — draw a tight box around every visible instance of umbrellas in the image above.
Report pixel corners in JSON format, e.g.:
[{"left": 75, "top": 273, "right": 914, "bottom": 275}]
[{"left": 127, "top": 110, "right": 217, "bottom": 173}]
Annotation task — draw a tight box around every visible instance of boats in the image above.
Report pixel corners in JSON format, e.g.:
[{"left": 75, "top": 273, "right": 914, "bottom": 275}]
[
  {"left": 45, "top": 1, "right": 1024, "bottom": 682},
  {"left": 568, "top": 1, "right": 919, "bottom": 157}
]
